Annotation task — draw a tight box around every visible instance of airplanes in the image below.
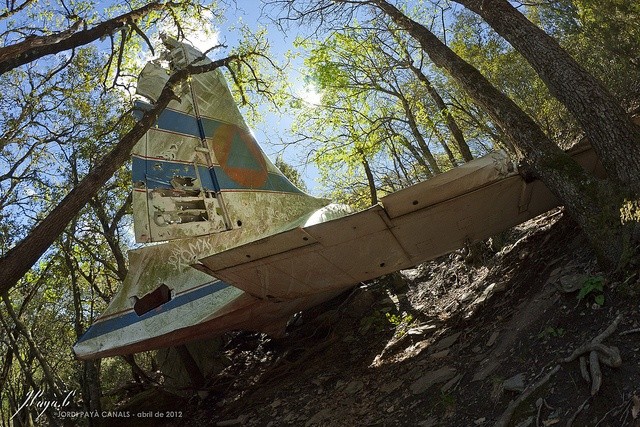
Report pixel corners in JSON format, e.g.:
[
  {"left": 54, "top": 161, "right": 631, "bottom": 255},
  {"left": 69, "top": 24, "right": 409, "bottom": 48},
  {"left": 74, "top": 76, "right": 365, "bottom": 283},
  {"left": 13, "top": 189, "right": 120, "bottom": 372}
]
[{"left": 45, "top": 18, "right": 624, "bottom": 394}]
